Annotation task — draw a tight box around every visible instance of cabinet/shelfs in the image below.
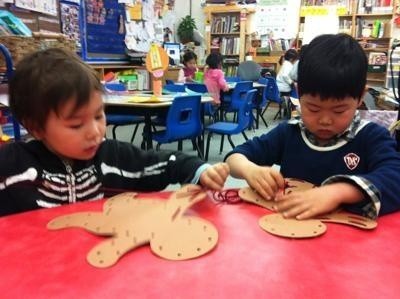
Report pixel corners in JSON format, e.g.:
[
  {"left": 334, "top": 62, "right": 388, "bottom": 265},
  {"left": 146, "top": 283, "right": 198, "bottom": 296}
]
[
  {"left": 203, "top": 0, "right": 255, "bottom": 77},
  {"left": 348, "top": 0, "right": 394, "bottom": 88}
]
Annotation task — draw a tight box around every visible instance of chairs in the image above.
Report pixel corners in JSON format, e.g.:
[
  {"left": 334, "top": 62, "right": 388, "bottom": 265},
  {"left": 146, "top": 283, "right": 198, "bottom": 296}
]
[{"left": 103, "top": 62, "right": 300, "bottom": 161}]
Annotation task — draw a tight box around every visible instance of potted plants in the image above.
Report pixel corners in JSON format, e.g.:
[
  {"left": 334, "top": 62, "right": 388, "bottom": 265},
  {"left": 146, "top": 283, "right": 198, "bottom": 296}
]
[{"left": 178, "top": 15, "right": 197, "bottom": 44}]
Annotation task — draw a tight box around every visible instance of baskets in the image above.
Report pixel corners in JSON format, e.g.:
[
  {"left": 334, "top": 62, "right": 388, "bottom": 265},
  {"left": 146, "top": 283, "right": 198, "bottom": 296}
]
[{"left": 0, "top": 32, "right": 77, "bottom": 67}]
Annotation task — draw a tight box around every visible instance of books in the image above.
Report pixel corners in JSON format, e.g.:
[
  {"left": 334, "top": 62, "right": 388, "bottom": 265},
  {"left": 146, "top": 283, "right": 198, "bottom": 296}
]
[
  {"left": 210, "top": 15, "right": 238, "bottom": 78},
  {"left": 59, "top": 1, "right": 180, "bottom": 91},
  {"left": 249, "top": 0, "right": 400, "bottom": 89}
]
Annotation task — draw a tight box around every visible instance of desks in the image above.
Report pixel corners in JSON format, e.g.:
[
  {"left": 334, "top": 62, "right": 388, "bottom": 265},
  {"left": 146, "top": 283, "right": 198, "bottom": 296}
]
[
  {"left": 0, "top": 188, "right": 400, "bottom": 299},
  {"left": 246, "top": 51, "right": 285, "bottom": 74}
]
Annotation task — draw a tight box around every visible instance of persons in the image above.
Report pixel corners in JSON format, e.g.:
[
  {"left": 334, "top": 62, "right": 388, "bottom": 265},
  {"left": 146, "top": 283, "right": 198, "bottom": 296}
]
[
  {"left": 202, "top": 51, "right": 232, "bottom": 107},
  {"left": 274, "top": 49, "right": 298, "bottom": 96},
  {"left": 182, "top": 51, "right": 198, "bottom": 82},
  {"left": 223, "top": 33, "right": 400, "bottom": 219},
  {"left": 1, "top": 47, "right": 230, "bottom": 218}
]
